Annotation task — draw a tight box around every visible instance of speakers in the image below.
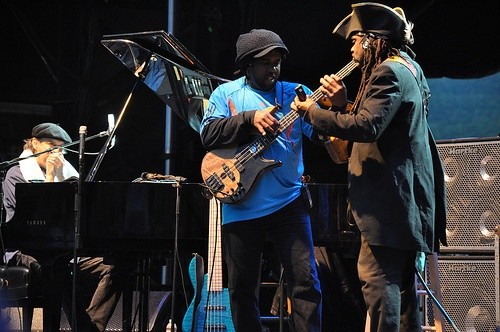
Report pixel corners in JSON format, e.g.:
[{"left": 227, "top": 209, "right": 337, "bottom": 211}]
[
  {"left": 424, "top": 254, "right": 500, "bottom": 332},
  {"left": 433, "top": 136, "right": 500, "bottom": 255},
  {"left": 58, "top": 289, "right": 181, "bottom": 332}
]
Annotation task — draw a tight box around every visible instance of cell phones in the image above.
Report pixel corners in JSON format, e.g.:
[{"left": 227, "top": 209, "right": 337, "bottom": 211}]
[{"left": 294, "top": 84, "right": 305, "bottom": 102}]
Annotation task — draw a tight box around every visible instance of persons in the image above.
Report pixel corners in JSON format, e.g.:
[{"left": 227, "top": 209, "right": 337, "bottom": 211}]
[
  {"left": 290, "top": 3, "right": 448, "bottom": 332},
  {"left": 1, "top": 124, "right": 123, "bottom": 332},
  {"left": 200, "top": 29, "right": 350, "bottom": 332}
]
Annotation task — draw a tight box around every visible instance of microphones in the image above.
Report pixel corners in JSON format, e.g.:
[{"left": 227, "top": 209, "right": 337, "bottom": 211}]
[
  {"left": 78, "top": 125, "right": 87, "bottom": 160},
  {"left": 107, "top": 113, "right": 115, "bottom": 150},
  {"left": 141, "top": 171, "right": 186, "bottom": 182}
]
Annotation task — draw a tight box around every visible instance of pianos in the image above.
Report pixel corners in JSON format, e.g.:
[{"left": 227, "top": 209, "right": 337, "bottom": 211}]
[{"left": 0, "top": 31, "right": 361, "bottom": 332}]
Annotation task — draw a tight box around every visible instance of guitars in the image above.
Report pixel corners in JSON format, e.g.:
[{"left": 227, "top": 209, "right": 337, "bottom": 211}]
[
  {"left": 201, "top": 60, "right": 360, "bottom": 203},
  {"left": 182, "top": 195, "right": 236, "bottom": 332}
]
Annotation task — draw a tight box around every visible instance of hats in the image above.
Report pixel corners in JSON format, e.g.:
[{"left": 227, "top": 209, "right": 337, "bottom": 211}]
[
  {"left": 235, "top": 29, "right": 290, "bottom": 64},
  {"left": 32, "top": 123, "right": 72, "bottom": 143},
  {"left": 332, "top": 2, "right": 414, "bottom": 44}
]
